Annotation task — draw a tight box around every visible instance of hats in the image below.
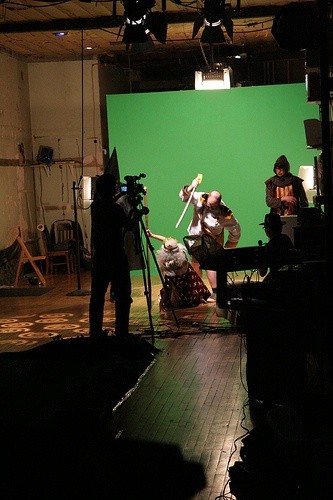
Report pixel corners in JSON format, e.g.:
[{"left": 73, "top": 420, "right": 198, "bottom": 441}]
[
  {"left": 258, "top": 213, "right": 287, "bottom": 227},
  {"left": 272, "top": 154, "right": 290, "bottom": 175}
]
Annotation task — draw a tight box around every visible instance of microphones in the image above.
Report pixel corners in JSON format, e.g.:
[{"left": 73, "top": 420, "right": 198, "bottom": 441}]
[{"left": 139, "top": 173, "right": 147, "bottom": 179}]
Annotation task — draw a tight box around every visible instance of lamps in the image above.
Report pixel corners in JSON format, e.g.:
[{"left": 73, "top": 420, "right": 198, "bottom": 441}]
[
  {"left": 201, "top": 3, "right": 225, "bottom": 43},
  {"left": 123, "top": 6, "right": 147, "bottom": 43}
]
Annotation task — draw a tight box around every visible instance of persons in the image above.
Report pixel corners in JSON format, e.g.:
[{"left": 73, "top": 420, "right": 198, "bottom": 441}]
[
  {"left": 146, "top": 229, "right": 211, "bottom": 312},
  {"left": 179, "top": 174, "right": 241, "bottom": 303},
  {"left": 255, "top": 213, "right": 295, "bottom": 276},
  {"left": 264, "top": 155, "right": 309, "bottom": 216},
  {"left": 89, "top": 174, "right": 149, "bottom": 337}
]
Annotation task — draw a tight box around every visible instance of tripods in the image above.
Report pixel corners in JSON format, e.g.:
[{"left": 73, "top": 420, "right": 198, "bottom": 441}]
[{"left": 126, "top": 195, "right": 181, "bottom": 345}]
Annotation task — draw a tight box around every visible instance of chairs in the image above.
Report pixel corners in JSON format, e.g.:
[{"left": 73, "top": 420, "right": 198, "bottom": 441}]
[
  {"left": 41, "top": 220, "right": 74, "bottom": 275},
  {"left": 14, "top": 236, "right": 48, "bottom": 286}
]
